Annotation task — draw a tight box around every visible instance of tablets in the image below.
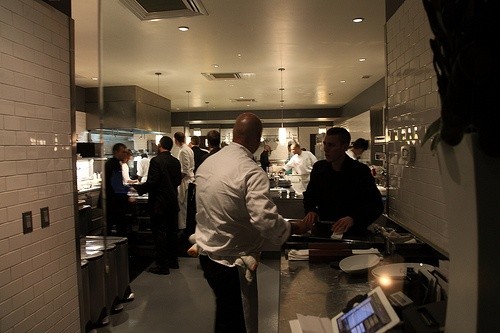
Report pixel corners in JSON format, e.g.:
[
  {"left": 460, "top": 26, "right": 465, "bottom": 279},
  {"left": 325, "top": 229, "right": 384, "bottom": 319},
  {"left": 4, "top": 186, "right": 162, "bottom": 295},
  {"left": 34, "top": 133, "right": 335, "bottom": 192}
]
[{"left": 331, "top": 287, "right": 400, "bottom": 333}]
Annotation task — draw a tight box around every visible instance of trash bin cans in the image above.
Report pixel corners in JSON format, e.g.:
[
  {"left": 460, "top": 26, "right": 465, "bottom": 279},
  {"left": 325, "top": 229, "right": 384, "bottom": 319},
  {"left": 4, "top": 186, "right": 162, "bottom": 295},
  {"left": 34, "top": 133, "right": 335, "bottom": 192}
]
[
  {"left": 81, "top": 260, "right": 97, "bottom": 333},
  {"left": 86, "top": 236, "right": 135, "bottom": 302},
  {"left": 81, "top": 250, "right": 109, "bottom": 326},
  {"left": 86, "top": 243, "right": 124, "bottom": 315}
]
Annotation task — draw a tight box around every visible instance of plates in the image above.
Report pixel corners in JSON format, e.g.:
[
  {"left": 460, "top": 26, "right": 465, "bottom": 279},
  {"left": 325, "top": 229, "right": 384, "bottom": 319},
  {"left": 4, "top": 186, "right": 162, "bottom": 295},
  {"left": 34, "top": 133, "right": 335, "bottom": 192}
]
[
  {"left": 81, "top": 236, "right": 127, "bottom": 242},
  {"left": 79, "top": 242, "right": 116, "bottom": 266},
  {"left": 370, "top": 262, "right": 435, "bottom": 279},
  {"left": 338, "top": 253, "right": 379, "bottom": 272}
]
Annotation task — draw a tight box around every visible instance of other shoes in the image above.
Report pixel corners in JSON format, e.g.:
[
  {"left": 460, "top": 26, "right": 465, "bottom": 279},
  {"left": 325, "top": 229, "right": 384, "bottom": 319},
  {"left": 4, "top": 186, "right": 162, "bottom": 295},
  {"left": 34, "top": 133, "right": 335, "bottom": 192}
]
[
  {"left": 157, "top": 263, "right": 179, "bottom": 269},
  {"left": 150, "top": 267, "right": 169, "bottom": 275}
]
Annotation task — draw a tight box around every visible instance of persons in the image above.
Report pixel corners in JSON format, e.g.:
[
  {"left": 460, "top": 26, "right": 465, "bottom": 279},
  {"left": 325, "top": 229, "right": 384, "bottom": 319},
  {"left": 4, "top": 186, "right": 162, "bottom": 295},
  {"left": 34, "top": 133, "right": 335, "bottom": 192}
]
[
  {"left": 303, "top": 127, "right": 385, "bottom": 235},
  {"left": 104, "top": 130, "right": 222, "bottom": 275},
  {"left": 277, "top": 141, "right": 317, "bottom": 195},
  {"left": 344, "top": 137, "right": 370, "bottom": 160},
  {"left": 194, "top": 112, "right": 312, "bottom": 333},
  {"left": 260, "top": 145, "right": 272, "bottom": 172}
]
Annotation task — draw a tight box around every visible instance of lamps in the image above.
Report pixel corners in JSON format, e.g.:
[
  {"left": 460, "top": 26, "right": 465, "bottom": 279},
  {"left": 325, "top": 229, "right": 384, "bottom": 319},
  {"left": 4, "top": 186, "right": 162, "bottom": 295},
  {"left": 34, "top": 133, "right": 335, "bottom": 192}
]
[
  {"left": 155, "top": 73, "right": 163, "bottom": 146},
  {"left": 186, "top": 90, "right": 192, "bottom": 145},
  {"left": 278, "top": 68, "right": 286, "bottom": 143}
]
